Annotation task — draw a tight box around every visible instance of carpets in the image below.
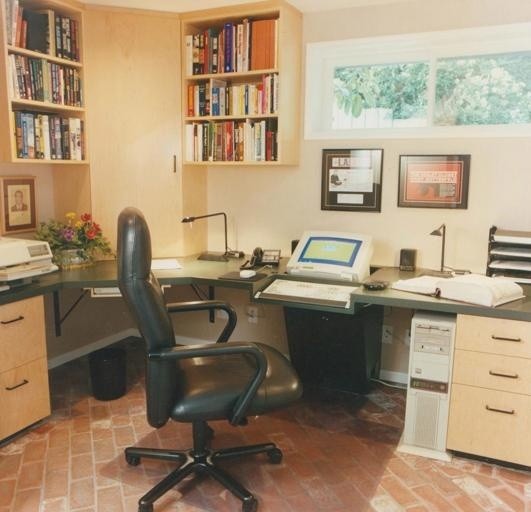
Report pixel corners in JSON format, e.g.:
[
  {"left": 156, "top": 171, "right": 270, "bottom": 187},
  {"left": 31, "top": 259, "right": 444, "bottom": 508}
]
[{"left": 99, "top": 399, "right": 399, "bottom": 511}]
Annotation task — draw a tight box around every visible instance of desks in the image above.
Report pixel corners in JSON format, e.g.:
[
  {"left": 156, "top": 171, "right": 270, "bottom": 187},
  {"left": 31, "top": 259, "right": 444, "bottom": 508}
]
[{"left": 0, "top": 251, "right": 531, "bottom": 474}]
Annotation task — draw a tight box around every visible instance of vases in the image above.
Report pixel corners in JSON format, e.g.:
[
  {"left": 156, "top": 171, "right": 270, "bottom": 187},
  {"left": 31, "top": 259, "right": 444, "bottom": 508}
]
[{"left": 52, "top": 248, "right": 93, "bottom": 264}]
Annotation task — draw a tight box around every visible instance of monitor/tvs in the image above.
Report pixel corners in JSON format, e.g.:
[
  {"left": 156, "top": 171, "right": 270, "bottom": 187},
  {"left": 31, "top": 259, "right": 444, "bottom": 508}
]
[{"left": 286, "top": 229, "right": 373, "bottom": 286}]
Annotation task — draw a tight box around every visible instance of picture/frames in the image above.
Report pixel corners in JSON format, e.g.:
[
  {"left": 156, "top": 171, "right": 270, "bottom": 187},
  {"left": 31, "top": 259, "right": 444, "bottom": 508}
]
[
  {"left": 398, "top": 153, "right": 470, "bottom": 209},
  {"left": 321, "top": 148, "right": 383, "bottom": 212},
  {"left": 0, "top": 176, "right": 38, "bottom": 236}
]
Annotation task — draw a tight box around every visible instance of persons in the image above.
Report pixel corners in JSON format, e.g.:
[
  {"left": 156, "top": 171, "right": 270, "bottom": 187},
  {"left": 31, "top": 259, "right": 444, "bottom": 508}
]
[
  {"left": 330, "top": 170, "right": 342, "bottom": 185},
  {"left": 10, "top": 191, "right": 28, "bottom": 212}
]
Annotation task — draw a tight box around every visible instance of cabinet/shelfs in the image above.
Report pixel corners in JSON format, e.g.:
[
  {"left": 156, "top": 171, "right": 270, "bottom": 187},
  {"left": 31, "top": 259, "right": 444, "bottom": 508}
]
[
  {"left": 0, "top": 0, "right": 90, "bottom": 165},
  {"left": 181, "top": 0, "right": 302, "bottom": 167},
  {"left": 487, "top": 226, "right": 531, "bottom": 288},
  {"left": 54, "top": 4, "right": 208, "bottom": 259}
]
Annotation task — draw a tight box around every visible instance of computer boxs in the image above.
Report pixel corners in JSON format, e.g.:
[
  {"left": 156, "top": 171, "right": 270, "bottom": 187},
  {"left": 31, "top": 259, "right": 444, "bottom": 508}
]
[{"left": 396, "top": 310, "right": 455, "bottom": 462}]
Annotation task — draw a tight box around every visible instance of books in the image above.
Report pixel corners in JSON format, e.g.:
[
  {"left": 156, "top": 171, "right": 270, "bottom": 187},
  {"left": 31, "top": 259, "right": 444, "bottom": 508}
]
[
  {"left": 184, "top": 16, "right": 280, "bottom": 76},
  {"left": 8, "top": 109, "right": 84, "bottom": 160},
  {"left": 6, "top": 53, "right": 85, "bottom": 108},
  {"left": 185, "top": 74, "right": 279, "bottom": 117},
  {"left": 391, "top": 273, "right": 526, "bottom": 308},
  {"left": 184, "top": 119, "right": 278, "bottom": 161},
  {"left": 4, "top": 0, "right": 82, "bottom": 64}
]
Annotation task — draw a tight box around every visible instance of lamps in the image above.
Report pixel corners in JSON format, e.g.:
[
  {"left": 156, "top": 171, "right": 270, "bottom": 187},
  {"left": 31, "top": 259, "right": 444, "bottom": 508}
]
[
  {"left": 181, "top": 213, "right": 242, "bottom": 262},
  {"left": 418, "top": 224, "right": 453, "bottom": 278}
]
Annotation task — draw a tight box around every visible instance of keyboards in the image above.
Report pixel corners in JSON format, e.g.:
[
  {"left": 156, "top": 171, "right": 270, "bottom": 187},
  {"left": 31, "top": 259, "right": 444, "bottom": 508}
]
[{"left": 255, "top": 279, "right": 359, "bottom": 310}]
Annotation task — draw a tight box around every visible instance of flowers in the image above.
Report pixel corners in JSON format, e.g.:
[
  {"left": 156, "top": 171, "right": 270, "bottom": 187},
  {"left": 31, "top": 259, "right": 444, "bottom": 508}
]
[{"left": 33, "top": 211, "right": 116, "bottom": 263}]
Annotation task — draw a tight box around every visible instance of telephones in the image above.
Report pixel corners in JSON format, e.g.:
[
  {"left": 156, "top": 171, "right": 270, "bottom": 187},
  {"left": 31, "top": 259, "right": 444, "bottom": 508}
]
[{"left": 240, "top": 247, "right": 280, "bottom": 270}]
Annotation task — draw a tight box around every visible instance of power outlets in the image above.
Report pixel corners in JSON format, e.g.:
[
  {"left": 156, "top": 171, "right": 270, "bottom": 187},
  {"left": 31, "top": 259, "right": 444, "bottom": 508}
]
[
  {"left": 248, "top": 306, "right": 258, "bottom": 323},
  {"left": 382, "top": 324, "right": 393, "bottom": 345}
]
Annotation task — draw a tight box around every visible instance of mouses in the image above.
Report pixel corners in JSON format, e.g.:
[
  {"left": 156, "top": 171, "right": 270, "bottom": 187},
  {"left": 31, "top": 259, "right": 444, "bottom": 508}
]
[{"left": 238, "top": 269, "right": 257, "bottom": 279}]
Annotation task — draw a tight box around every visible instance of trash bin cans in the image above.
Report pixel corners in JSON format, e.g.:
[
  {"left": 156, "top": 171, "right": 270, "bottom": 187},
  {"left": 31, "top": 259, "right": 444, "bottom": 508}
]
[{"left": 90, "top": 347, "right": 127, "bottom": 401}]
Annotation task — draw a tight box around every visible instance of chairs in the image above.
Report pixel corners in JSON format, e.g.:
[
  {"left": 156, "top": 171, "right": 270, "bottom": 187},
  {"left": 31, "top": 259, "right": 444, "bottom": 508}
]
[{"left": 117, "top": 207, "right": 304, "bottom": 512}]
